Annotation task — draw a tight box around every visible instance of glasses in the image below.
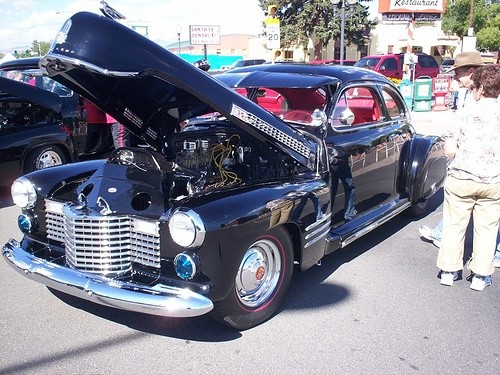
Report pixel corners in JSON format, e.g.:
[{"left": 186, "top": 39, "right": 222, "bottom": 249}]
[{"left": 470, "top": 84, "right": 480, "bottom": 90}]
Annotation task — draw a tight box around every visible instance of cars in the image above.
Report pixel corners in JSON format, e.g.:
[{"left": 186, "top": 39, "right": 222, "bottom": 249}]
[
  {"left": 231, "top": 51, "right": 457, "bottom": 81},
  {"left": 1, "top": 0, "right": 448, "bottom": 332},
  {"left": 0, "top": 76, "right": 75, "bottom": 194}
]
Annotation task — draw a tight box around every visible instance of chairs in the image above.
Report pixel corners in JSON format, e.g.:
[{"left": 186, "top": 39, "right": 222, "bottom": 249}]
[{"left": 285, "top": 111, "right": 366, "bottom": 129}]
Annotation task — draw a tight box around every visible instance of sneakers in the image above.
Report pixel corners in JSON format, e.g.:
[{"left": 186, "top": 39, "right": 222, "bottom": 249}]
[
  {"left": 470, "top": 273, "right": 492, "bottom": 291},
  {"left": 419, "top": 225, "right": 441, "bottom": 249},
  {"left": 440, "top": 271, "right": 458, "bottom": 287}
]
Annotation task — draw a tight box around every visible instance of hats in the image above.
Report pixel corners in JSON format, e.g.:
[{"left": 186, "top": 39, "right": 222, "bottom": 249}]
[{"left": 445, "top": 52, "right": 487, "bottom": 74}]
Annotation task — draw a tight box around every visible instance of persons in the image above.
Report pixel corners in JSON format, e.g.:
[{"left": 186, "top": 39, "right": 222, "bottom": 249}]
[
  {"left": 79, "top": 97, "right": 130, "bottom": 162},
  {"left": 325, "top": 139, "right": 358, "bottom": 216},
  {"left": 293, "top": 188, "right": 326, "bottom": 226},
  {"left": 418, "top": 51, "right": 499, "bottom": 291},
  {"left": 26, "top": 73, "right": 36, "bottom": 86},
  {"left": 266, "top": 199, "right": 295, "bottom": 229}
]
